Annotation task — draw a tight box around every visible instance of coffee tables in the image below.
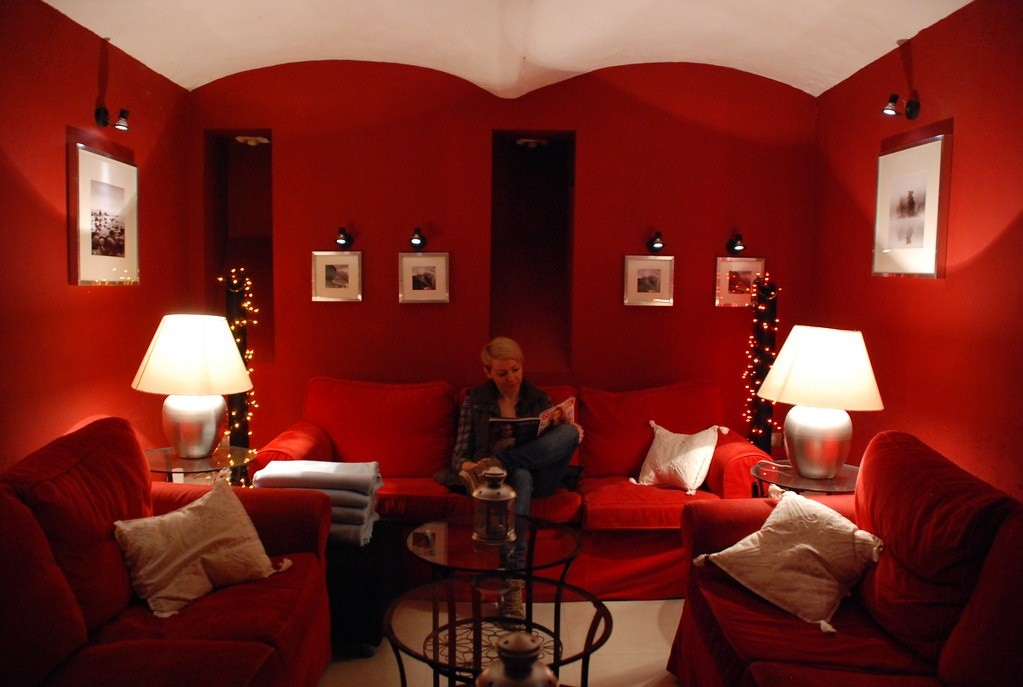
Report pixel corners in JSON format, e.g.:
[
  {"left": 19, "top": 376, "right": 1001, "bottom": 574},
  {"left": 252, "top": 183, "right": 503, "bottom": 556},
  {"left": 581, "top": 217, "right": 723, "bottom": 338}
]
[{"left": 377, "top": 519, "right": 613, "bottom": 687}]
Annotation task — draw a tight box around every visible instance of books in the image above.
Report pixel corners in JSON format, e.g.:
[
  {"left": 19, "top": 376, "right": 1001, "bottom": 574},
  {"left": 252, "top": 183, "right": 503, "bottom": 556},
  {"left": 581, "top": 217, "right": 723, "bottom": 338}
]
[{"left": 487, "top": 396, "right": 574, "bottom": 458}]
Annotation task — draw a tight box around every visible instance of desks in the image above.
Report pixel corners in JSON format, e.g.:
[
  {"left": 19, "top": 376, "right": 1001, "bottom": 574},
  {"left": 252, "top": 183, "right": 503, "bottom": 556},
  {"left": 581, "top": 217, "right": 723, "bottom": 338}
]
[{"left": 143, "top": 444, "right": 255, "bottom": 486}]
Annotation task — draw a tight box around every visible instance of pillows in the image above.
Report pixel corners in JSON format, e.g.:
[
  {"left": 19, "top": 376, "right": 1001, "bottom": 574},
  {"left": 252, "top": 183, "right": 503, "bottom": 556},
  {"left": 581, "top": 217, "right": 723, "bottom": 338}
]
[
  {"left": 113, "top": 467, "right": 291, "bottom": 620},
  {"left": 628, "top": 419, "right": 730, "bottom": 496},
  {"left": 707, "top": 488, "right": 884, "bottom": 631}
]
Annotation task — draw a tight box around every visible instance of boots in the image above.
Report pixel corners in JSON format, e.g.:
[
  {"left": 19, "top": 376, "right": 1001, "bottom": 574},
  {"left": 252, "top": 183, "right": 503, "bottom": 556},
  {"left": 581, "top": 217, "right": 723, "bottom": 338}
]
[
  {"left": 497, "top": 575, "right": 525, "bottom": 630},
  {"left": 460, "top": 456, "right": 501, "bottom": 497}
]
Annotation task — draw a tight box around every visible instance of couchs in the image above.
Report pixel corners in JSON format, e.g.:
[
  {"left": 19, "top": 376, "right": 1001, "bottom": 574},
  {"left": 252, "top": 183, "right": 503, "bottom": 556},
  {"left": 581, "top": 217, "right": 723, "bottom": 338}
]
[
  {"left": 664, "top": 430, "right": 1023, "bottom": 687},
  {"left": 244, "top": 378, "right": 777, "bottom": 605},
  {"left": 1, "top": 415, "right": 337, "bottom": 686}
]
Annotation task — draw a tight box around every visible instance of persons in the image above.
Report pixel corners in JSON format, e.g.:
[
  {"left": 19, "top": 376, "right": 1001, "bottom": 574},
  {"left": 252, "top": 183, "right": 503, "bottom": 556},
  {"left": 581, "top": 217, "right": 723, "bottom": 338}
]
[
  {"left": 728, "top": 272, "right": 744, "bottom": 294},
  {"left": 413, "top": 272, "right": 436, "bottom": 290},
  {"left": 326, "top": 265, "right": 349, "bottom": 287},
  {"left": 896, "top": 189, "right": 915, "bottom": 243},
  {"left": 539, "top": 407, "right": 566, "bottom": 436},
  {"left": 638, "top": 276, "right": 660, "bottom": 292},
  {"left": 493, "top": 422, "right": 516, "bottom": 453},
  {"left": 91, "top": 226, "right": 125, "bottom": 257},
  {"left": 451, "top": 338, "right": 585, "bottom": 630}
]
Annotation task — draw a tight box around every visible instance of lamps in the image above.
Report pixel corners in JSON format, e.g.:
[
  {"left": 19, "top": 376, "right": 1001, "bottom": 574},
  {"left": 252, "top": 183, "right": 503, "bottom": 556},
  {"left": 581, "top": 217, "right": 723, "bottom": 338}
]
[
  {"left": 648, "top": 232, "right": 663, "bottom": 253},
  {"left": 131, "top": 312, "right": 254, "bottom": 459},
  {"left": 757, "top": 324, "right": 885, "bottom": 478},
  {"left": 410, "top": 227, "right": 425, "bottom": 248},
  {"left": 727, "top": 235, "right": 744, "bottom": 254},
  {"left": 882, "top": 93, "right": 919, "bottom": 119},
  {"left": 95, "top": 106, "right": 130, "bottom": 131},
  {"left": 336, "top": 227, "right": 352, "bottom": 248}
]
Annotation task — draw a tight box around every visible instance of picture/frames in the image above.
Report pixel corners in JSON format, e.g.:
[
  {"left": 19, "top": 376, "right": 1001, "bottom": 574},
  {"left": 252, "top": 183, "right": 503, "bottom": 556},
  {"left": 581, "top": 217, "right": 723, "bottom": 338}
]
[
  {"left": 67, "top": 141, "right": 141, "bottom": 287},
  {"left": 311, "top": 251, "right": 363, "bottom": 303},
  {"left": 397, "top": 252, "right": 451, "bottom": 304},
  {"left": 870, "top": 133, "right": 951, "bottom": 280},
  {"left": 715, "top": 257, "right": 765, "bottom": 308},
  {"left": 624, "top": 255, "right": 674, "bottom": 307}
]
